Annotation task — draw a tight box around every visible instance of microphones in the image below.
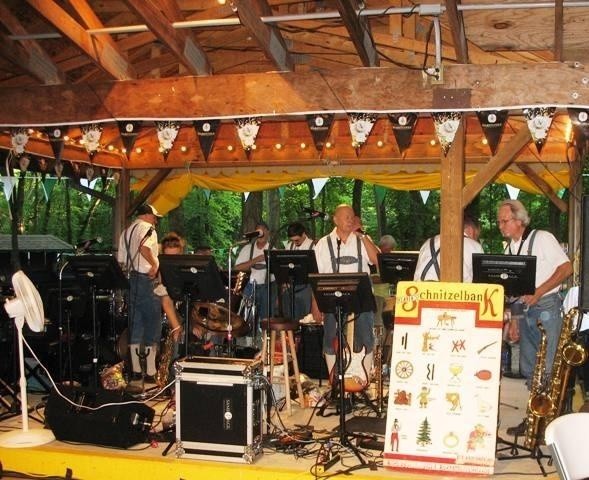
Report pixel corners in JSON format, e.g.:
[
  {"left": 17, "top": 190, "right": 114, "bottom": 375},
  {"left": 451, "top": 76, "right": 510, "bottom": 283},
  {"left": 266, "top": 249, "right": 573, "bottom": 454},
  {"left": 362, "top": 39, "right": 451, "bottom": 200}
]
[
  {"left": 74, "top": 236, "right": 104, "bottom": 250},
  {"left": 303, "top": 207, "right": 329, "bottom": 221},
  {"left": 243, "top": 229, "right": 263, "bottom": 239},
  {"left": 356, "top": 228, "right": 367, "bottom": 235}
]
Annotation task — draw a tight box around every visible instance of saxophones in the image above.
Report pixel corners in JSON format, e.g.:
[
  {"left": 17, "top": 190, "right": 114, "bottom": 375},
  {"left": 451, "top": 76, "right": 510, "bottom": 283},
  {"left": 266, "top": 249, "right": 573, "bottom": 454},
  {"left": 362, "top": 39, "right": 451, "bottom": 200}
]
[
  {"left": 524, "top": 317, "right": 554, "bottom": 448},
  {"left": 541, "top": 308, "right": 587, "bottom": 446}
]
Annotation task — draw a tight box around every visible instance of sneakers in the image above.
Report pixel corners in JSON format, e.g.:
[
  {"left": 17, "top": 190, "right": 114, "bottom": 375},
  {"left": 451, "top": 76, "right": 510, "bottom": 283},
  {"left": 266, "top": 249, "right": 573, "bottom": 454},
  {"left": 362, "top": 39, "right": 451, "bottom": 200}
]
[{"left": 130, "top": 371, "right": 159, "bottom": 389}]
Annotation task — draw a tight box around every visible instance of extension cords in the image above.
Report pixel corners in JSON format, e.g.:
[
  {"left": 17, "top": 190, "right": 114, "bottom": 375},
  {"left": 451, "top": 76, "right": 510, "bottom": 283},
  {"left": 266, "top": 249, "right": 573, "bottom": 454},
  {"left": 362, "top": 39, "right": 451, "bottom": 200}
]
[{"left": 316, "top": 455, "right": 340, "bottom": 473}]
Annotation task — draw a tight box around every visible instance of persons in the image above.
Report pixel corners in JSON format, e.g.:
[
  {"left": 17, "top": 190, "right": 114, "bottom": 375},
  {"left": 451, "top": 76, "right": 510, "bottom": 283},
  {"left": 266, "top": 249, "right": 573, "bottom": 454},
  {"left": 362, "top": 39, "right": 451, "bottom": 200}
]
[
  {"left": 158, "top": 204, "right": 316, "bottom": 357},
  {"left": 563, "top": 286, "right": 589, "bottom": 413},
  {"left": 311, "top": 204, "right": 383, "bottom": 381},
  {"left": 117, "top": 204, "right": 164, "bottom": 384},
  {"left": 372, "top": 235, "right": 396, "bottom": 337},
  {"left": 413, "top": 210, "right": 484, "bottom": 282},
  {"left": 497, "top": 200, "right": 573, "bottom": 436}
]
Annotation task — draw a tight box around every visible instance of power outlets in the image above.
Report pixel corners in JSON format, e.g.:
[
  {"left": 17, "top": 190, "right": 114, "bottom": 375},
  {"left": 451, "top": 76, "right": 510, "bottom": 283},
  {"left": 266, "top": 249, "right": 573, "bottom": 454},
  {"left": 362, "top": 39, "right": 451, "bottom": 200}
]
[{"left": 431, "top": 64, "right": 444, "bottom": 85}]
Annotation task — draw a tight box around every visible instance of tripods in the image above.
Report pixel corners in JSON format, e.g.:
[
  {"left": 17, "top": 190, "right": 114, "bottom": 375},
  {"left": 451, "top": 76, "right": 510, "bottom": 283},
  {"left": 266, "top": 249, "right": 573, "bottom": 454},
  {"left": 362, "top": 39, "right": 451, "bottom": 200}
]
[
  {"left": 314, "top": 306, "right": 377, "bottom": 467},
  {"left": 498, "top": 416, "right": 551, "bottom": 477}
]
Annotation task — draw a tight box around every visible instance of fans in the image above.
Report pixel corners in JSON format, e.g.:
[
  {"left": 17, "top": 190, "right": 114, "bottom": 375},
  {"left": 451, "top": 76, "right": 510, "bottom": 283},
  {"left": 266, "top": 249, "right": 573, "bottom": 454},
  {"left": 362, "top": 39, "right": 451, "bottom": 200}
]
[{"left": 0, "top": 271, "right": 55, "bottom": 448}]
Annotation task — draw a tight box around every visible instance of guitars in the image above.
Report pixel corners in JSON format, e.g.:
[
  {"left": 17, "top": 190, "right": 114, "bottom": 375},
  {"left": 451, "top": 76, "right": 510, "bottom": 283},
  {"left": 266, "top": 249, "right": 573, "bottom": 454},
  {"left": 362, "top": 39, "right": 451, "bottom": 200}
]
[{"left": 329, "top": 312, "right": 369, "bottom": 393}]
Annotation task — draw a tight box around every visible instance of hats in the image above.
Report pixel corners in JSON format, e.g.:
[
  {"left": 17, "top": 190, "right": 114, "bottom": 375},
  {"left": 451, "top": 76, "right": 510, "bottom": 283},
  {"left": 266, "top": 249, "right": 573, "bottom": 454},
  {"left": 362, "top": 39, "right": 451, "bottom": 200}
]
[{"left": 138, "top": 204, "right": 164, "bottom": 218}]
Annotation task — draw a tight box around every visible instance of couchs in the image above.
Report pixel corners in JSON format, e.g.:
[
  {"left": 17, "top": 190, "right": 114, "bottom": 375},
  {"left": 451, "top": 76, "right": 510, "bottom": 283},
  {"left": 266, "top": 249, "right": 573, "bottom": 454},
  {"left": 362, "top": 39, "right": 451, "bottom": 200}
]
[{"left": 258, "top": 317, "right": 304, "bottom": 416}]
